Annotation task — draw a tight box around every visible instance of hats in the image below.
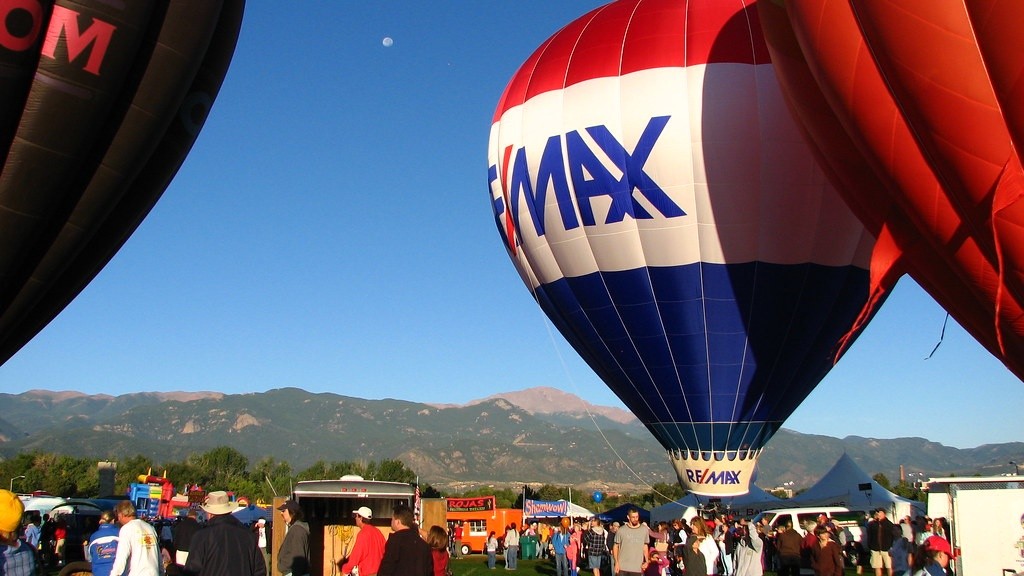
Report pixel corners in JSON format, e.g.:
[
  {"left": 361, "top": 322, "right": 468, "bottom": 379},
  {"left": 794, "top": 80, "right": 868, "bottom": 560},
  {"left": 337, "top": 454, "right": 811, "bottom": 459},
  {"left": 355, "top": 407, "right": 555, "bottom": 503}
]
[
  {"left": 277, "top": 500, "right": 299, "bottom": 511},
  {"left": 613, "top": 522, "right": 620, "bottom": 527},
  {"left": 353, "top": 507, "right": 372, "bottom": 519},
  {"left": 198, "top": 491, "right": 232, "bottom": 515},
  {"left": 875, "top": 507, "right": 886, "bottom": 514},
  {"left": 924, "top": 535, "right": 956, "bottom": 560},
  {"left": 0, "top": 489, "right": 24, "bottom": 533}
]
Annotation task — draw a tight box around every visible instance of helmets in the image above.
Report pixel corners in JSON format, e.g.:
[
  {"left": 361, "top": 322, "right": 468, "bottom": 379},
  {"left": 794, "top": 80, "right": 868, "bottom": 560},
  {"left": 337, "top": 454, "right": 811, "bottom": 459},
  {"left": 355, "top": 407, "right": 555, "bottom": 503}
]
[{"left": 101, "top": 510, "right": 115, "bottom": 520}]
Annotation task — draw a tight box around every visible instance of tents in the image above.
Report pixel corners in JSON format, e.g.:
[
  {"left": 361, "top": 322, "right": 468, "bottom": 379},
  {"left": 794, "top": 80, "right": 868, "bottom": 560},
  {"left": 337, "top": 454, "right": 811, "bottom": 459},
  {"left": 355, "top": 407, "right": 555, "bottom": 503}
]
[
  {"left": 0, "top": 489, "right": 960, "bottom": 576},
  {"left": 594, "top": 503, "right": 650, "bottom": 527},
  {"left": 522, "top": 498, "right": 595, "bottom": 528},
  {"left": 649, "top": 461, "right": 785, "bottom": 527},
  {"left": 785, "top": 450, "right": 925, "bottom": 524}
]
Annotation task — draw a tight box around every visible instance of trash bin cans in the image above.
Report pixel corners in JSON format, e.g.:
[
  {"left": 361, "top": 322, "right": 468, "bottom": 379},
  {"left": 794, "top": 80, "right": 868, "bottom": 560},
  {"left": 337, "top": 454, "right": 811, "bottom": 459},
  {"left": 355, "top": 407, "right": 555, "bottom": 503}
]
[{"left": 519, "top": 535, "right": 538, "bottom": 561}]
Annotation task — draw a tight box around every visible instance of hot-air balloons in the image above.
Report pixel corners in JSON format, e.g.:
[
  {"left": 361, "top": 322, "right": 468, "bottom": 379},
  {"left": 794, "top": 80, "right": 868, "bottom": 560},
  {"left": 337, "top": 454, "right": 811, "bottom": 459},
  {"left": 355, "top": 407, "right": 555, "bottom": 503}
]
[
  {"left": 0, "top": 0, "right": 247, "bottom": 364},
  {"left": 487, "top": 0, "right": 907, "bottom": 529},
  {"left": 757, "top": 0, "right": 1024, "bottom": 384}
]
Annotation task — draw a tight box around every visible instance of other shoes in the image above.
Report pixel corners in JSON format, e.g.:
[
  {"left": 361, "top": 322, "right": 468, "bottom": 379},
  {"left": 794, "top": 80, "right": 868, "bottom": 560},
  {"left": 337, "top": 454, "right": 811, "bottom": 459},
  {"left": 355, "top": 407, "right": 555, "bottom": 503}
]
[{"left": 490, "top": 566, "right": 496, "bottom": 569}]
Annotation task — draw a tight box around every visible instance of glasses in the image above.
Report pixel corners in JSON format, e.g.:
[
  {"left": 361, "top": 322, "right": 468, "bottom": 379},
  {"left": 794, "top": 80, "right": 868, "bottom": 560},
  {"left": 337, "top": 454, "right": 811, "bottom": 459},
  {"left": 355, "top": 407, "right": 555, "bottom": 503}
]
[
  {"left": 657, "top": 525, "right": 661, "bottom": 526},
  {"left": 874, "top": 510, "right": 879, "bottom": 513},
  {"left": 672, "top": 523, "right": 678, "bottom": 527}
]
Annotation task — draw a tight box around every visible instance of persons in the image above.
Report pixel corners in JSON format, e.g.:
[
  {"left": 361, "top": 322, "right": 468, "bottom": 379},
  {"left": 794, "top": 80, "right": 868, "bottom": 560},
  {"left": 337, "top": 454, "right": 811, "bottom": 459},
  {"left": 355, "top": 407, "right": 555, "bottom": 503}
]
[
  {"left": 341, "top": 506, "right": 387, "bottom": 576},
  {"left": 277, "top": 500, "right": 310, "bottom": 576},
  {"left": 164, "top": 491, "right": 267, "bottom": 576},
  {"left": 377, "top": 506, "right": 435, "bottom": 576},
  {"left": 426, "top": 525, "right": 450, "bottom": 576}
]
